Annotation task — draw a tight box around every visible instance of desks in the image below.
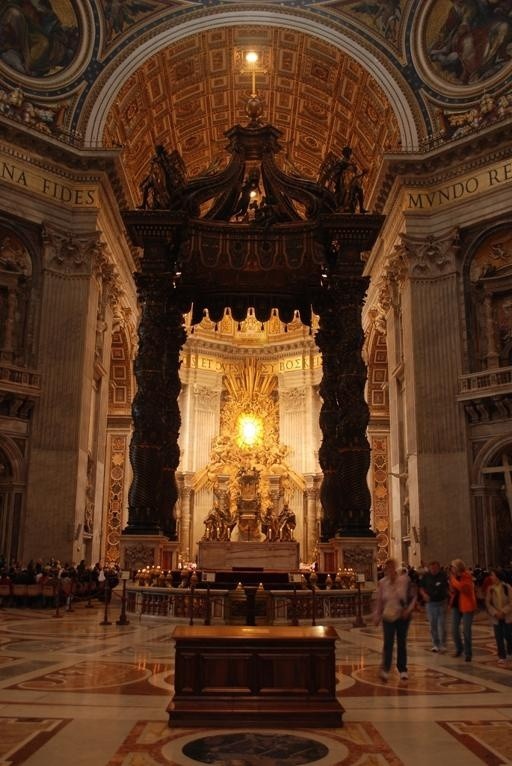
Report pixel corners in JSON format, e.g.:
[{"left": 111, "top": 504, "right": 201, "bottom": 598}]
[{"left": 166, "top": 625, "right": 345, "bottom": 727}]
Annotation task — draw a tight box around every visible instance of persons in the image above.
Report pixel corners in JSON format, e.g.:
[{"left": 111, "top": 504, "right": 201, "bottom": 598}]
[{"left": 135, "top": 144, "right": 182, "bottom": 210}]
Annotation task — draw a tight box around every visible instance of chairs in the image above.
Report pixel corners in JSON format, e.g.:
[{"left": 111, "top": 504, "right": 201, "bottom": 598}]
[{"left": 0, "top": 581, "right": 105, "bottom": 609}]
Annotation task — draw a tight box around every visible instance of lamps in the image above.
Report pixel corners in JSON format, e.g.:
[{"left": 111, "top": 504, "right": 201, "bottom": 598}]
[{"left": 245, "top": 52, "right": 258, "bottom": 98}]
